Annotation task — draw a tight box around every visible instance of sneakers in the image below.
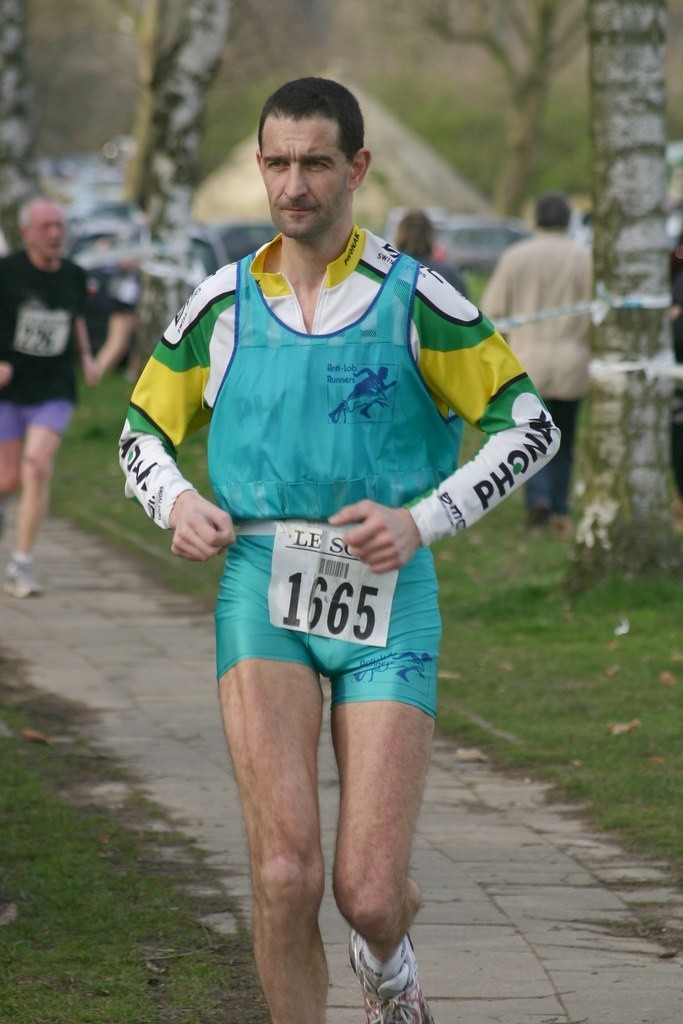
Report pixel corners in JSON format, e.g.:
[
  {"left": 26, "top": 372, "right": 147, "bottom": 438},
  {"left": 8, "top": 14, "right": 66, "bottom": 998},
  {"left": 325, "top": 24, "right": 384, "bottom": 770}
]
[{"left": 348, "top": 927, "right": 436, "bottom": 1024}]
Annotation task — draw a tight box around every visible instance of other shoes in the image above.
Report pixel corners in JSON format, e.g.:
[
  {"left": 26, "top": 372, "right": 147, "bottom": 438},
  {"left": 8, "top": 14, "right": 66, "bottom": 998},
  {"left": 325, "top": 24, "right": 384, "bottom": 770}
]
[
  {"left": 4, "top": 558, "right": 43, "bottom": 599},
  {"left": 526, "top": 497, "right": 553, "bottom": 527}
]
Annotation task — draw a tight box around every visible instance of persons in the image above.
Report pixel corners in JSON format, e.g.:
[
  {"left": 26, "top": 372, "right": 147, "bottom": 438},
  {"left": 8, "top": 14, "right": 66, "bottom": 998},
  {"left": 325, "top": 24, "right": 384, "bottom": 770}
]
[
  {"left": 1, "top": 202, "right": 149, "bottom": 598},
  {"left": 118, "top": 77, "right": 564, "bottom": 1024},
  {"left": 483, "top": 194, "right": 593, "bottom": 534},
  {"left": 396, "top": 209, "right": 470, "bottom": 296}
]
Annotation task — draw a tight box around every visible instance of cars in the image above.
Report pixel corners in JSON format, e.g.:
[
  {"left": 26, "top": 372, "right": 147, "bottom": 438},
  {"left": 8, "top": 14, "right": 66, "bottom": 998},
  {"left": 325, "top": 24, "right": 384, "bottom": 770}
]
[
  {"left": 435, "top": 212, "right": 533, "bottom": 274},
  {"left": 167, "top": 222, "right": 281, "bottom": 312}
]
